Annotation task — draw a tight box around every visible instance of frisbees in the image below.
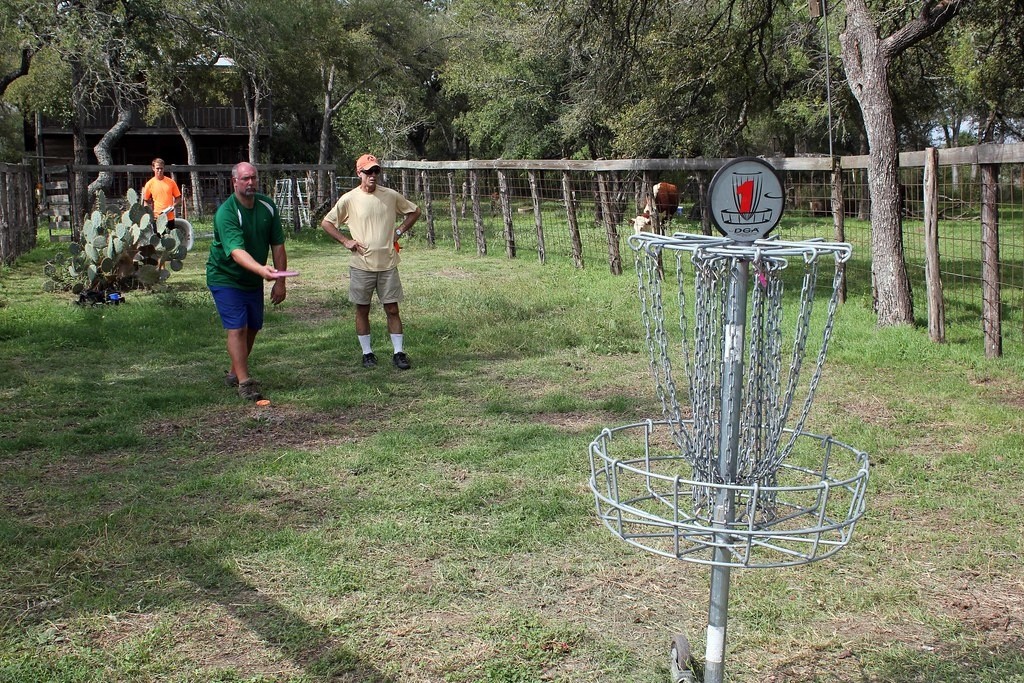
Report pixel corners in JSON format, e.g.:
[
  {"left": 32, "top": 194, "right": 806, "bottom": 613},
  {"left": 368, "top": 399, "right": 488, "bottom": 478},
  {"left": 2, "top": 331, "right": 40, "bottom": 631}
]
[{"left": 271, "top": 271, "right": 301, "bottom": 277}]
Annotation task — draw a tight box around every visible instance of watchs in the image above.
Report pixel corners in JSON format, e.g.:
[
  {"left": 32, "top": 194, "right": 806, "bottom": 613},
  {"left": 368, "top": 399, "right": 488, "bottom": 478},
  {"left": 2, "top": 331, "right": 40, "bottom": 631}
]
[{"left": 395, "top": 228, "right": 402, "bottom": 236}]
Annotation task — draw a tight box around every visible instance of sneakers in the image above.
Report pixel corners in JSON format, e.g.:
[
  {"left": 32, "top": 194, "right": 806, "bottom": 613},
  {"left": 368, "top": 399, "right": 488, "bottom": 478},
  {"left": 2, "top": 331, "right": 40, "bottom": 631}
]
[
  {"left": 392, "top": 352, "right": 410, "bottom": 369},
  {"left": 225, "top": 375, "right": 259, "bottom": 387},
  {"left": 238, "top": 379, "right": 262, "bottom": 399},
  {"left": 363, "top": 353, "right": 378, "bottom": 367}
]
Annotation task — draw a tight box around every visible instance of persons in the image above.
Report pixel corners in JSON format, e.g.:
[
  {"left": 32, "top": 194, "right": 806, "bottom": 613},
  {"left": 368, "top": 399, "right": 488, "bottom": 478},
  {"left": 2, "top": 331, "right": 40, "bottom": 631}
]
[
  {"left": 205, "top": 161, "right": 287, "bottom": 402},
  {"left": 321, "top": 153, "right": 421, "bottom": 370},
  {"left": 144, "top": 158, "right": 182, "bottom": 268}
]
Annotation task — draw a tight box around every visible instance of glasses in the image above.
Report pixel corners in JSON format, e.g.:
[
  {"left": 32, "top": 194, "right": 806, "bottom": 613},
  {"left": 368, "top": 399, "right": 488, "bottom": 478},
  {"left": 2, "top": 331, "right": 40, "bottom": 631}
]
[{"left": 361, "top": 168, "right": 380, "bottom": 175}]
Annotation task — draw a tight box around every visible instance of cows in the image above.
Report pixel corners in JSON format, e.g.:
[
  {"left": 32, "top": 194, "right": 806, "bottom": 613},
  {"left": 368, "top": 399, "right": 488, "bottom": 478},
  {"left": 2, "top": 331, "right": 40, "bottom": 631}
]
[
  {"left": 625, "top": 183, "right": 678, "bottom": 235},
  {"left": 490, "top": 193, "right": 510, "bottom": 218}
]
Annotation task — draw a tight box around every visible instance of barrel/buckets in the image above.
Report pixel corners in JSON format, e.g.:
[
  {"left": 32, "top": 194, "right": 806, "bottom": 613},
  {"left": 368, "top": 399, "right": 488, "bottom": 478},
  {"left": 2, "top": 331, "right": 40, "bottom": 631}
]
[{"left": 677, "top": 206, "right": 683, "bottom": 216}]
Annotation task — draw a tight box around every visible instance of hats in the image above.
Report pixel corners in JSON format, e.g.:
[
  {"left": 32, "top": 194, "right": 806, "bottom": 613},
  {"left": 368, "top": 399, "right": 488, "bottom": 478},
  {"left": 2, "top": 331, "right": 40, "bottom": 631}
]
[{"left": 356, "top": 154, "right": 380, "bottom": 171}]
